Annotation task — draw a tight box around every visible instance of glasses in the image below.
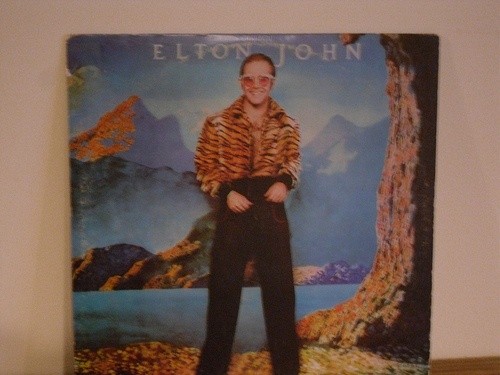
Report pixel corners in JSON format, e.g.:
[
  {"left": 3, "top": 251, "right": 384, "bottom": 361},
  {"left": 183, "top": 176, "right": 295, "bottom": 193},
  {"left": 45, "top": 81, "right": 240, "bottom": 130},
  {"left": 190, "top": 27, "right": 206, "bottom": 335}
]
[{"left": 237, "top": 72, "right": 277, "bottom": 87}]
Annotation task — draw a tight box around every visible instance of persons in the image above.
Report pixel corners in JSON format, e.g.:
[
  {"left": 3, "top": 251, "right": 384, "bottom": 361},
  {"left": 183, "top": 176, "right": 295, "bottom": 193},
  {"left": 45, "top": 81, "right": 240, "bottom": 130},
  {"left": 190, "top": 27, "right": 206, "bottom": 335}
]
[{"left": 193, "top": 52, "right": 301, "bottom": 375}]
